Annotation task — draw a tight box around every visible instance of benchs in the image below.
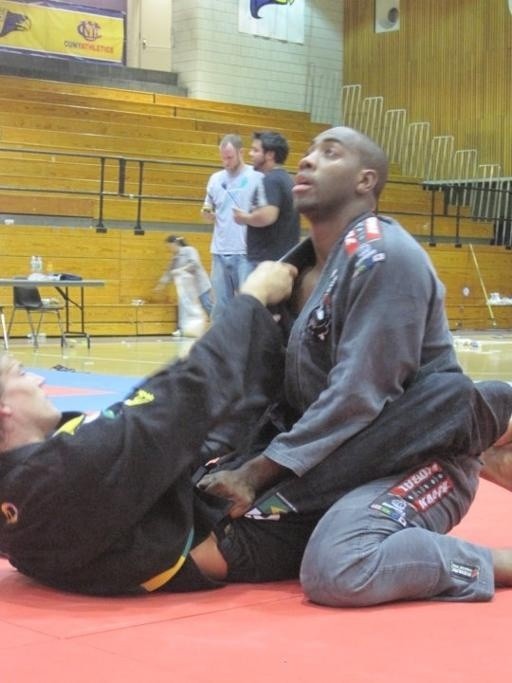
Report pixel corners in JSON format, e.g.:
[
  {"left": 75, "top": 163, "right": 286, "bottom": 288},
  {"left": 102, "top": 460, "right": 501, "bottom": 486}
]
[{"left": 0, "top": 305, "right": 64, "bottom": 350}]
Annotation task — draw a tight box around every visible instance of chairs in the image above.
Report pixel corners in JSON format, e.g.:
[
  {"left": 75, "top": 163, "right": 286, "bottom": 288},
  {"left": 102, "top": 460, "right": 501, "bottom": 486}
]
[{"left": 7, "top": 274, "right": 70, "bottom": 348}]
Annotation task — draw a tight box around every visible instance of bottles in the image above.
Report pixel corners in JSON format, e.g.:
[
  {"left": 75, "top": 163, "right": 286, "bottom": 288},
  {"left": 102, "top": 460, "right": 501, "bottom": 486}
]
[{"left": 29, "top": 254, "right": 43, "bottom": 281}]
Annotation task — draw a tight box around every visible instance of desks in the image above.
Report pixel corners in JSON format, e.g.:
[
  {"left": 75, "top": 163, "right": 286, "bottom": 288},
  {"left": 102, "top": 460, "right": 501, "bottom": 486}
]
[{"left": 0, "top": 279, "right": 106, "bottom": 349}]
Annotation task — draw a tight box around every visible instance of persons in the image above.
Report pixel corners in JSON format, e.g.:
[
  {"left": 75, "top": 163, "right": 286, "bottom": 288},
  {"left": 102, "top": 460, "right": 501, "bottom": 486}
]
[
  {"left": 196, "top": 126, "right": 512, "bottom": 607},
  {"left": 1, "top": 261, "right": 512, "bottom": 597},
  {"left": 156, "top": 235, "right": 212, "bottom": 336},
  {"left": 202, "top": 134, "right": 265, "bottom": 300},
  {"left": 233, "top": 132, "right": 301, "bottom": 294}
]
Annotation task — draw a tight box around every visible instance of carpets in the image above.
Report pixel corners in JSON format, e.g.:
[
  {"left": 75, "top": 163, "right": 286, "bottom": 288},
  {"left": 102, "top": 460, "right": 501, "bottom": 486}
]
[{"left": 0, "top": 366, "right": 511, "bottom": 683}]
